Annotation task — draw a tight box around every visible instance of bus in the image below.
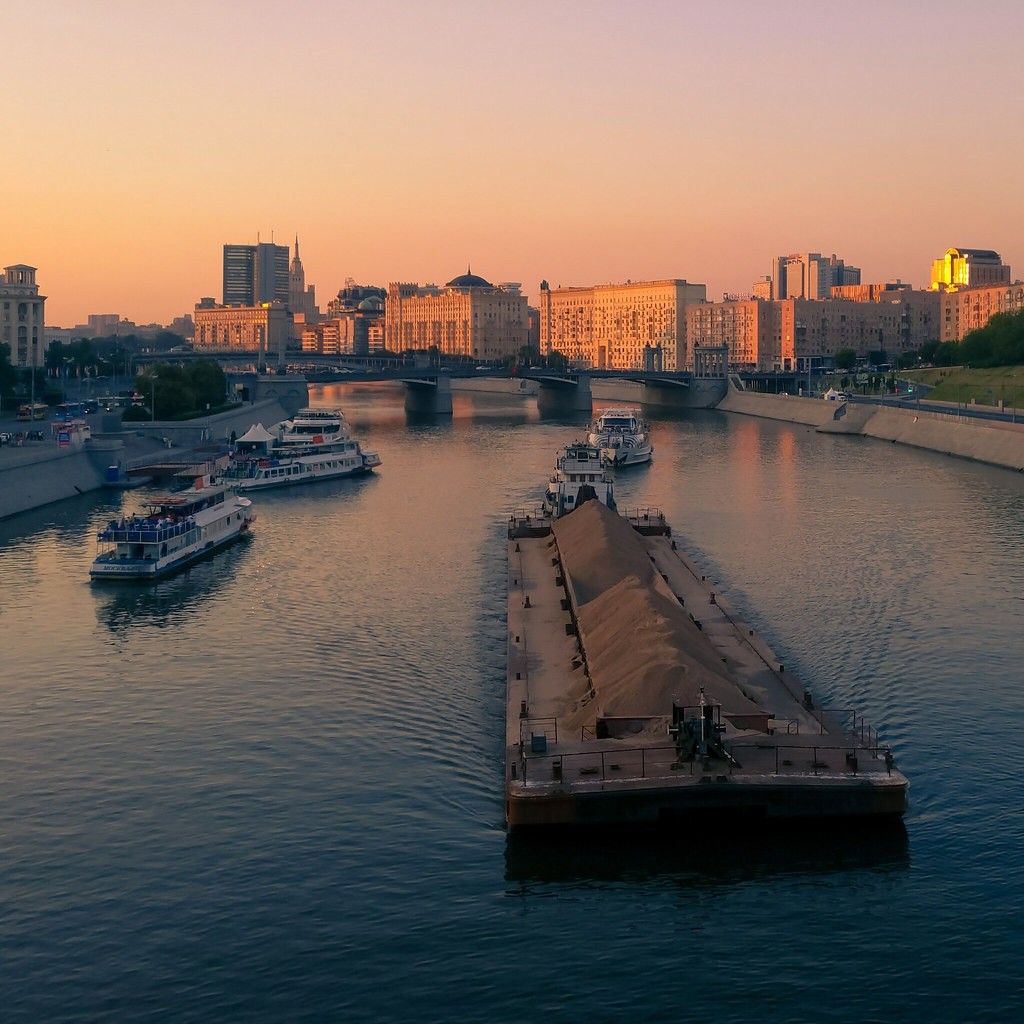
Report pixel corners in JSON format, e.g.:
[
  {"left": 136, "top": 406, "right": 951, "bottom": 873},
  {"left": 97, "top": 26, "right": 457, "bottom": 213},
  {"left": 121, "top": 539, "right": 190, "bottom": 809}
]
[
  {"left": 95, "top": 395, "right": 146, "bottom": 408},
  {"left": 82, "top": 398, "right": 99, "bottom": 414},
  {"left": 15, "top": 403, "right": 49, "bottom": 421},
  {"left": 54, "top": 401, "right": 86, "bottom": 422}
]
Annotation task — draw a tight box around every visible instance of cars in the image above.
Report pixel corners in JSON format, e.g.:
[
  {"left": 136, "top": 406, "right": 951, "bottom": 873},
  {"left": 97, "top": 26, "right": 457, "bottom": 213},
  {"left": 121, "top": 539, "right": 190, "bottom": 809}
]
[
  {"left": 530, "top": 365, "right": 543, "bottom": 371},
  {"left": 476, "top": 365, "right": 492, "bottom": 371},
  {"left": 440, "top": 366, "right": 453, "bottom": 373},
  {"left": 0, "top": 432, "right": 13, "bottom": 441}
]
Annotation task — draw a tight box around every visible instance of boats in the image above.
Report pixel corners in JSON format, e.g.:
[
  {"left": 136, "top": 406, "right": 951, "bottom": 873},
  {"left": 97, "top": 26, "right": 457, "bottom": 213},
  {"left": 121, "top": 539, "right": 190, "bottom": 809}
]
[
  {"left": 213, "top": 406, "right": 384, "bottom": 492},
  {"left": 542, "top": 439, "right": 616, "bottom": 519},
  {"left": 88, "top": 473, "right": 258, "bottom": 581},
  {"left": 582, "top": 403, "right": 655, "bottom": 469}
]
